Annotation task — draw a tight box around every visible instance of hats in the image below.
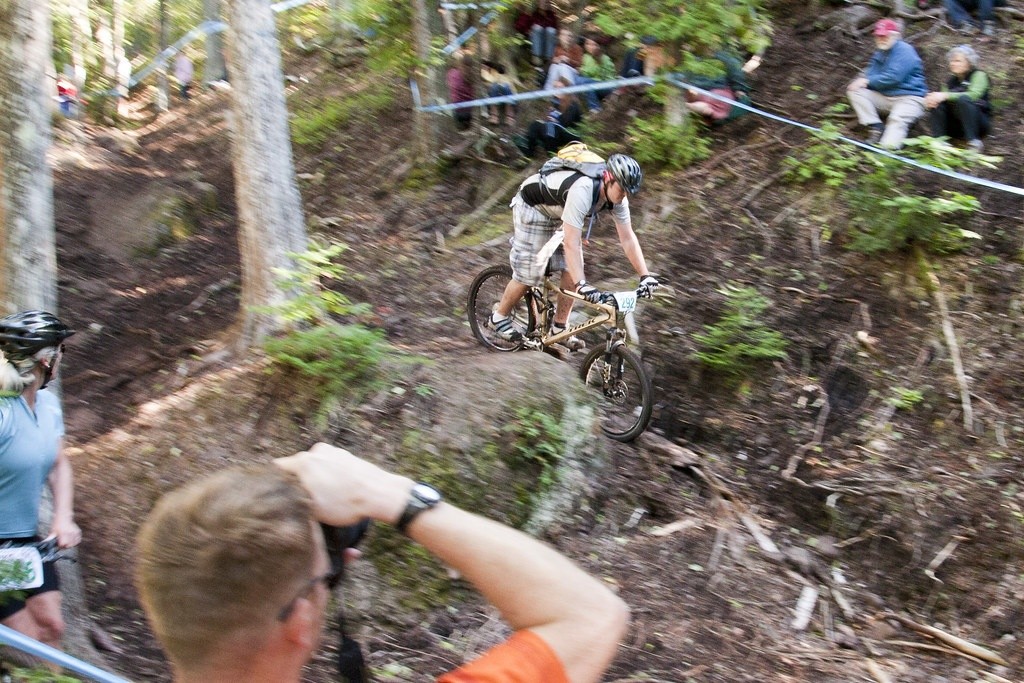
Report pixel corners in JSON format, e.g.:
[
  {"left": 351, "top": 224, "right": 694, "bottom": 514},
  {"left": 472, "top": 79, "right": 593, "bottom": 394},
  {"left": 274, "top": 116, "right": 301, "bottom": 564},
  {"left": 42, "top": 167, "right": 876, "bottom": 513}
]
[{"left": 871, "top": 18, "right": 900, "bottom": 36}]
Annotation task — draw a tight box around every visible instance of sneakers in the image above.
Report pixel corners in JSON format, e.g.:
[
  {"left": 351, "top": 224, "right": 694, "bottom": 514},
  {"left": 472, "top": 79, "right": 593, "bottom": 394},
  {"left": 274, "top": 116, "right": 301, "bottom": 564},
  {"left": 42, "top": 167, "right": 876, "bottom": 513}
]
[
  {"left": 487, "top": 314, "right": 522, "bottom": 341},
  {"left": 548, "top": 327, "right": 585, "bottom": 349}
]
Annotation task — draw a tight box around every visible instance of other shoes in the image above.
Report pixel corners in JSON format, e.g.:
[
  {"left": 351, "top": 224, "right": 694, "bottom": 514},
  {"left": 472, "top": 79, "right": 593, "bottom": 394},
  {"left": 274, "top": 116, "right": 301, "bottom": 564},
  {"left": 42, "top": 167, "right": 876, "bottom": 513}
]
[
  {"left": 982, "top": 20, "right": 996, "bottom": 37},
  {"left": 865, "top": 127, "right": 883, "bottom": 146},
  {"left": 965, "top": 143, "right": 986, "bottom": 156},
  {"left": 960, "top": 23, "right": 980, "bottom": 37}
]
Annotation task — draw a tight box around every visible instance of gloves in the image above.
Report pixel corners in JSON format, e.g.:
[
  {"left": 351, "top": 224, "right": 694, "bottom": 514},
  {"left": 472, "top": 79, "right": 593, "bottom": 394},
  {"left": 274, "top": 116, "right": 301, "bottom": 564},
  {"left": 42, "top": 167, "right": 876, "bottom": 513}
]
[
  {"left": 574, "top": 280, "right": 602, "bottom": 304},
  {"left": 636, "top": 275, "right": 658, "bottom": 299}
]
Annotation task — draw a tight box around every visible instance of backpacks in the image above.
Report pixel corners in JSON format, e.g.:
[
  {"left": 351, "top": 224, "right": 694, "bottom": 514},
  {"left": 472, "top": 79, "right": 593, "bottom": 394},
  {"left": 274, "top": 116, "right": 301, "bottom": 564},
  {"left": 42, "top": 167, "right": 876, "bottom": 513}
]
[{"left": 539, "top": 140, "right": 613, "bottom": 217}]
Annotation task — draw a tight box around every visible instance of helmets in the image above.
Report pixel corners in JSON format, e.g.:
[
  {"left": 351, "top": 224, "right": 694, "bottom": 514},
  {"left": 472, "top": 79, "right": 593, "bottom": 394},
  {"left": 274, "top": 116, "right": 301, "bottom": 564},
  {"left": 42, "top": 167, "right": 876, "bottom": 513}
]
[
  {"left": 0, "top": 310, "right": 69, "bottom": 360},
  {"left": 606, "top": 154, "right": 642, "bottom": 194}
]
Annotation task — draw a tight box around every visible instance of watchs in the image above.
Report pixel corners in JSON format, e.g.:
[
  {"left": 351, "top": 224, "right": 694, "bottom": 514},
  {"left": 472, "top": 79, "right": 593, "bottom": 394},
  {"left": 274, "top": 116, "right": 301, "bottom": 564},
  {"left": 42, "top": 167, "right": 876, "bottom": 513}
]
[
  {"left": 396, "top": 481, "right": 441, "bottom": 534},
  {"left": 575, "top": 279, "right": 586, "bottom": 290}
]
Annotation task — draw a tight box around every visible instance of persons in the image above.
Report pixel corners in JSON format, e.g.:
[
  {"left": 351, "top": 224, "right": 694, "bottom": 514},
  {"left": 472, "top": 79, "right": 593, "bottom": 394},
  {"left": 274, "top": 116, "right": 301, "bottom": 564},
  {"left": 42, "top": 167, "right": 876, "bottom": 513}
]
[
  {"left": 57, "top": 73, "right": 89, "bottom": 119},
  {"left": 138, "top": 442, "right": 631, "bottom": 683},
  {"left": 0, "top": 310, "right": 82, "bottom": 675},
  {"left": 448, "top": 0, "right": 750, "bottom": 163},
  {"left": 328, "top": 37, "right": 370, "bottom": 67},
  {"left": 488, "top": 152, "right": 658, "bottom": 351},
  {"left": 846, "top": 19, "right": 928, "bottom": 150},
  {"left": 944, "top": 0, "right": 996, "bottom": 35},
  {"left": 175, "top": 50, "right": 194, "bottom": 103},
  {"left": 924, "top": 46, "right": 992, "bottom": 153}
]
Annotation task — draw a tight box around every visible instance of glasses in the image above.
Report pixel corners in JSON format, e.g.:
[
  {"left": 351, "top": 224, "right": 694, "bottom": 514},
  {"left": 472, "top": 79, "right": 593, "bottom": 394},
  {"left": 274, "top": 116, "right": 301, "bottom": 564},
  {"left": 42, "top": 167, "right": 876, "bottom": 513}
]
[
  {"left": 276, "top": 548, "right": 345, "bottom": 622},
  {"left": 60, "top": 344, "right": 66, "bottom": 354}
]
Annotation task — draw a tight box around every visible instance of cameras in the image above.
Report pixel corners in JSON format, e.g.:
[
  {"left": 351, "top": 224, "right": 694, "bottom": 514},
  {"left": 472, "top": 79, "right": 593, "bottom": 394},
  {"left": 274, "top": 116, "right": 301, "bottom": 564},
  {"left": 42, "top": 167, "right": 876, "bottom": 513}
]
[{"left": 319, "top": 519, "right": 369, "bottom": 587}]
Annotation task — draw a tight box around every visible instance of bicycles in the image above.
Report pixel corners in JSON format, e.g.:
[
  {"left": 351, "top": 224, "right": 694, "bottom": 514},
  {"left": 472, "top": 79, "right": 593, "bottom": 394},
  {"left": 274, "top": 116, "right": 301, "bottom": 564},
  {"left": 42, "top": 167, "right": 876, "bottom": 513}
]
[
  {"left": 467, "top": 246, "right": 668, "bottom": 443},
  {"left": 0, "top": 534, "right": 77, "bottom": 597}
]
